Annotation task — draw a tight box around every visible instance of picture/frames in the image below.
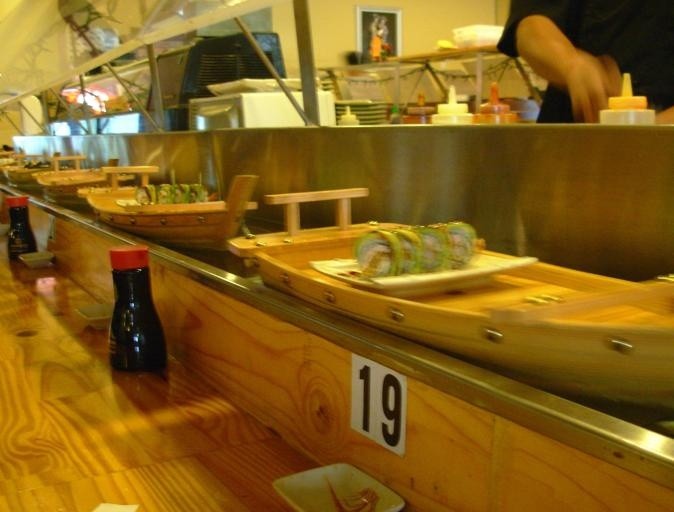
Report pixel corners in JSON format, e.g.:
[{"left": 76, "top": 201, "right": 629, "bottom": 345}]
[{"left": 357, "top": 4, "right": 402, "bottom": 62}]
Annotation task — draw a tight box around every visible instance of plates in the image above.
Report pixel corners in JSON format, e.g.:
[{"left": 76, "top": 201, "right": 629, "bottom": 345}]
[
  {"left": 112, "top": 199, "right": 152, "bottom": 208},
  {"left": 333, "top": 101, "right": 389, "bottom": 126},
  {"left": 16, "top": 252, "right": 56, "bottom": 270},
  {"left": 73, "top": 303, "right": 116, "bottom": 330},
  {"left": 269, "top": 461, "right": 407, "bottom": 512},
  {"left": 303, "top": 254, "right": 533, "bottom": 300},
  {"left": 206, "top": 75, "right": 320, "bottom": 96}
]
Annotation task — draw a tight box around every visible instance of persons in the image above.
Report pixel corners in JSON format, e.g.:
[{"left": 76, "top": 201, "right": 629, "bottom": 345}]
[
  {"left": 496, "top": 0, "right": 673, "bottom": 125},
  {"left": 368, "top": 13, "right": 389, "bottom": 61}
]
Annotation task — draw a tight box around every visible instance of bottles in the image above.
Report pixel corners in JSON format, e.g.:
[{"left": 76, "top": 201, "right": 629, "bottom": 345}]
[
  {"left": 431, "top": 86, "right": 474, "bottom": 124},
  {"left": 471, "top": 80, "right": 518, "bottom": 124},
  {"left": 104, "top": 244, "right": 167, "bottom": 372},
  {"left": 7, "top": 195, "right": 37, "bottom": 262},
  {"left": 402, "top": 92, "right": 434, "bottom": 125},
  {"left": 598, "top": 72, "right": 655, "bottom": 125}
]
